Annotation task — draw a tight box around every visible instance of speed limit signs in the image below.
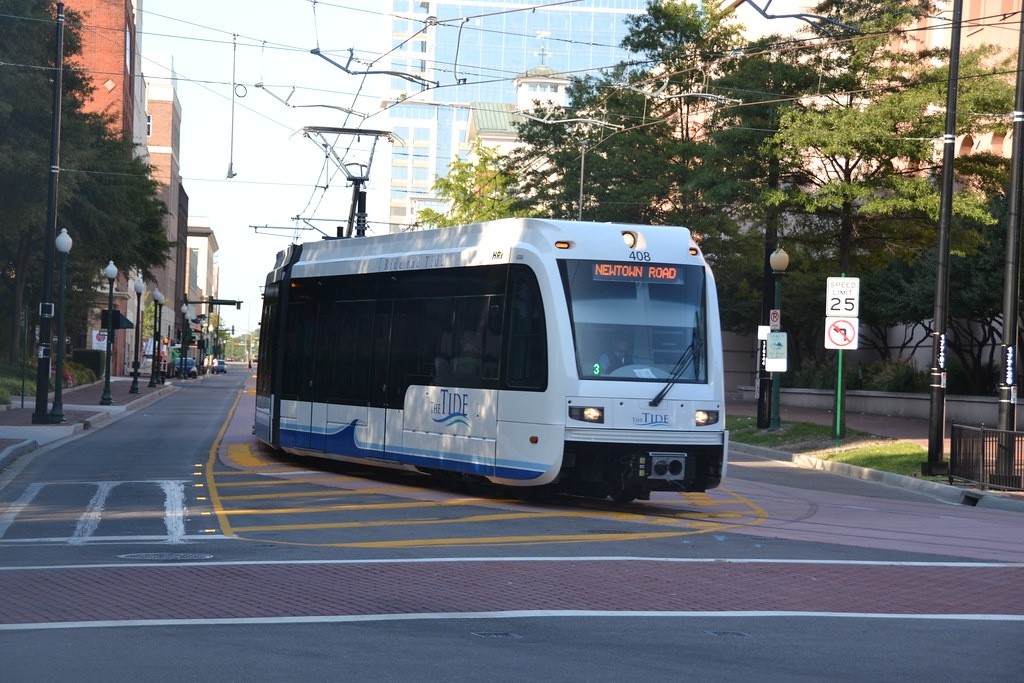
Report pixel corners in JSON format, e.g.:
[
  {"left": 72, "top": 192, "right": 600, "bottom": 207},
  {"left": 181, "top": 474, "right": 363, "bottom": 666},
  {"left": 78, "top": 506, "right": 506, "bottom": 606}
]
[{"left": 824, "top": 276, "right": 859, "bottom": 453}]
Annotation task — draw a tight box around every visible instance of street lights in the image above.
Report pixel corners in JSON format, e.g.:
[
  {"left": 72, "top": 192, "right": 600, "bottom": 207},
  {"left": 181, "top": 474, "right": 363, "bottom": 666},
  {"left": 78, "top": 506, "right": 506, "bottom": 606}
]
[
  {"left": 49, "top": 227, "right": 73, "bottom": 424},
  {"left": 97, "top": 259, "right": 118, "bottom": 406},
  {"left": 128, "top": 278, "right": 145, "bottom": 394},
  {"left": 179, "top": 302, "right": 187, "bottom": 379},
  {"left": 156, "top": 294, "right": 165, "bottom": 384},
  {"left": 184, "top": 310, "right": 191, "bottom": 379},
  {"left": 763, "top": 245, "right": 790, "bottom": 431},
  {"left": 147, "top": 287, "right": 161, "bottom": 388}
]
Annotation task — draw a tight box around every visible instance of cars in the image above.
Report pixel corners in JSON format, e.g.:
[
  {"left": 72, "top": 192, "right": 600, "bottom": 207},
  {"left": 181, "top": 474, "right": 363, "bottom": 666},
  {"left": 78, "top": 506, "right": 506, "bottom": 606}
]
[
  {"left": 173, "top": 358, "right": 198, "bottom": 380},
  {"left": 211, "top": 360, "right": 227, "bottom": 374}
]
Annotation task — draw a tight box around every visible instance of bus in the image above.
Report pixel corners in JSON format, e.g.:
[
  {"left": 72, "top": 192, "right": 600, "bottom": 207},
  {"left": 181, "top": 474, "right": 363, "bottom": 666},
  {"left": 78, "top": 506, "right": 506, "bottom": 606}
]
[{"left": 252, "top": 217, "right": 729, "bottom": 505}]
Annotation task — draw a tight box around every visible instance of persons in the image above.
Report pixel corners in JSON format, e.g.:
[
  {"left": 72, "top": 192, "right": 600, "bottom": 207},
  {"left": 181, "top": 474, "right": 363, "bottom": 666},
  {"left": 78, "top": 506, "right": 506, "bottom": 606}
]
[
  {"left": 190, "top": 357, "right": 254, "bottom": 375},
  {"left": 595, "top": 327, "right": 635, "bottom": 376},
  {"left": 430, "top": 314, "right": 497, "bottom": 390}
]
